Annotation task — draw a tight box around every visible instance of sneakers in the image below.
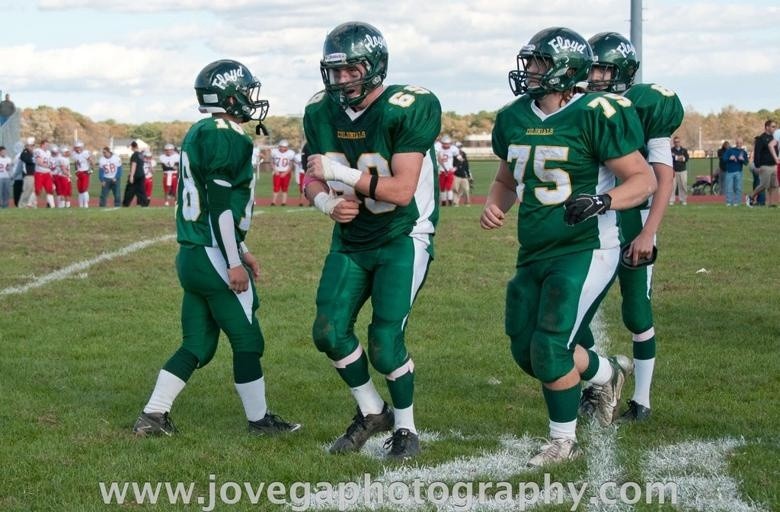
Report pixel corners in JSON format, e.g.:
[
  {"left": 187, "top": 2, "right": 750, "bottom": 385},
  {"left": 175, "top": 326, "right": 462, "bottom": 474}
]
[
  {"left": 746, "top": 194, "right": 754, "bottom": 207},
  {"left": 249, "top": 408, "right": 301, "bottom": 435},
  {"left": 528, "top": 437, "right": 582, "bottom": 467},
  {"left": 330, "top": 401, "right": 394, "bottom": 455},
  {"left": 132, "top": 410, "right": 182, "bottom": 438},
  {"left": 384, "top": 429, "right": 420, "bottom": 459},
  {"left": 578, "top": 355, "right": 652, "bottom": 429}
]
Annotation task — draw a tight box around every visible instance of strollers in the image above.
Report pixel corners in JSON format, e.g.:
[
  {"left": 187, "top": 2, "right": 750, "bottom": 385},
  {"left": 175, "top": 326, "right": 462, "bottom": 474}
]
[{"left": 692, "top": 167, "right": 721, "bottom": 196}]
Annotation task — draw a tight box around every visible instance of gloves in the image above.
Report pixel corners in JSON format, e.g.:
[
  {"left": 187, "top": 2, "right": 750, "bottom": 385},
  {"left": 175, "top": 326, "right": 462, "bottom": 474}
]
[{"left": 563, "top": 193, "right": 612, "bottom": 227}]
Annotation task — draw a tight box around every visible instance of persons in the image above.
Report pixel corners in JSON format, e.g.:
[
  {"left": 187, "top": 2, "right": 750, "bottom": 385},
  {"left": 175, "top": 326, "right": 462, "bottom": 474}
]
[
  {"left": 479, "top": 28, "right": 658, "bottom": 468},
  {"left": 577, "top": 33, "right": 684, "bottom": 424},
  {"left": 0, "top": 136, "right": 473, "bottom": 208},
  {"left": 301, "top": 23, "right": 440, "bottom": 461},
  {"left": 668, "top": 120, "right": 780, "bottom": 208},
  {"left": 132, "top": 60, "right": 301, "bottom": 439}
]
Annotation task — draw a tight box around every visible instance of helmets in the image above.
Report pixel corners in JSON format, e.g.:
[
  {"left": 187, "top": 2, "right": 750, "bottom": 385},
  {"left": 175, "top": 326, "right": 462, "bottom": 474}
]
[
  {"left": 194, "top": 59, "right": 260, "bottom": 121},
  {"left": 22, "top": 134, "right": 452, "bottom": 149},
  {"left": 519, "top": 28, "right": 637, "bottom": 98},
  {"left": 320, "top": 21, "right": 388, "bottom": 107}
]
